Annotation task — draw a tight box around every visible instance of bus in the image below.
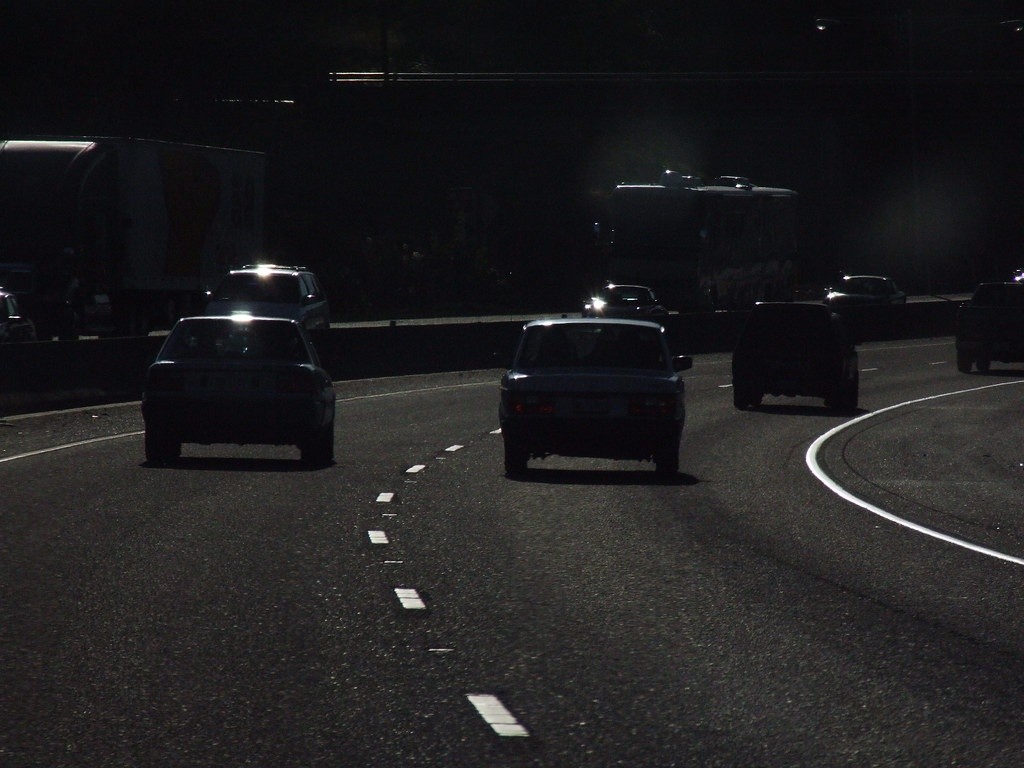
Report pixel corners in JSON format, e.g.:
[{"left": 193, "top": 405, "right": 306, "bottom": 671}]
[{"left": 591, "top": 172, "right": 801, "bottom": 302}]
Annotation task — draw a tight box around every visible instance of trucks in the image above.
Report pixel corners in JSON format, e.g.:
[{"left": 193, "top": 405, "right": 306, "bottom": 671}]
[{"left": 0, "top": 132, "right": 273, "bottom": 344}]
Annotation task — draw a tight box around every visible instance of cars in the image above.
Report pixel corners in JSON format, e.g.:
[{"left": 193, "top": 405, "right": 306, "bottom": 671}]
[
  {"left": 591, "top": 282, "right": 667, "bottom": 320},
  {"left": 0, "top": 286, "right": 42, "bottom": 345},
  {"left": 824, "top": 273, "right": 908, "bottom": 310},
  {"left": 499, "top": 317, "right": 692, "bottom": 477},
  {"left": 140, "top": 314, "right": 335, "bottom": 473},
  {"left": 954, "top": 279, "right": 1024, "bottom": 375}
]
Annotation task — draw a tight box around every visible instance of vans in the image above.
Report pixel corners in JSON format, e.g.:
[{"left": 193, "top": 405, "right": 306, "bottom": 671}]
[{"left": 207, "top": 261, "right": 332, "bottom": 322}]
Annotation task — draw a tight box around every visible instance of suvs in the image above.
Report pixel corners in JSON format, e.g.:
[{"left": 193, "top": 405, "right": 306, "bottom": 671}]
[{"left": 730, "top": 300, "right": 860, "bottom": 412}]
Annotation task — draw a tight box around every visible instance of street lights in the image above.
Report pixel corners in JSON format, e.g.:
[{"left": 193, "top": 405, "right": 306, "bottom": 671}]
[{"left": 814, "top": 8, "right": 922, "bottom": 293}]
